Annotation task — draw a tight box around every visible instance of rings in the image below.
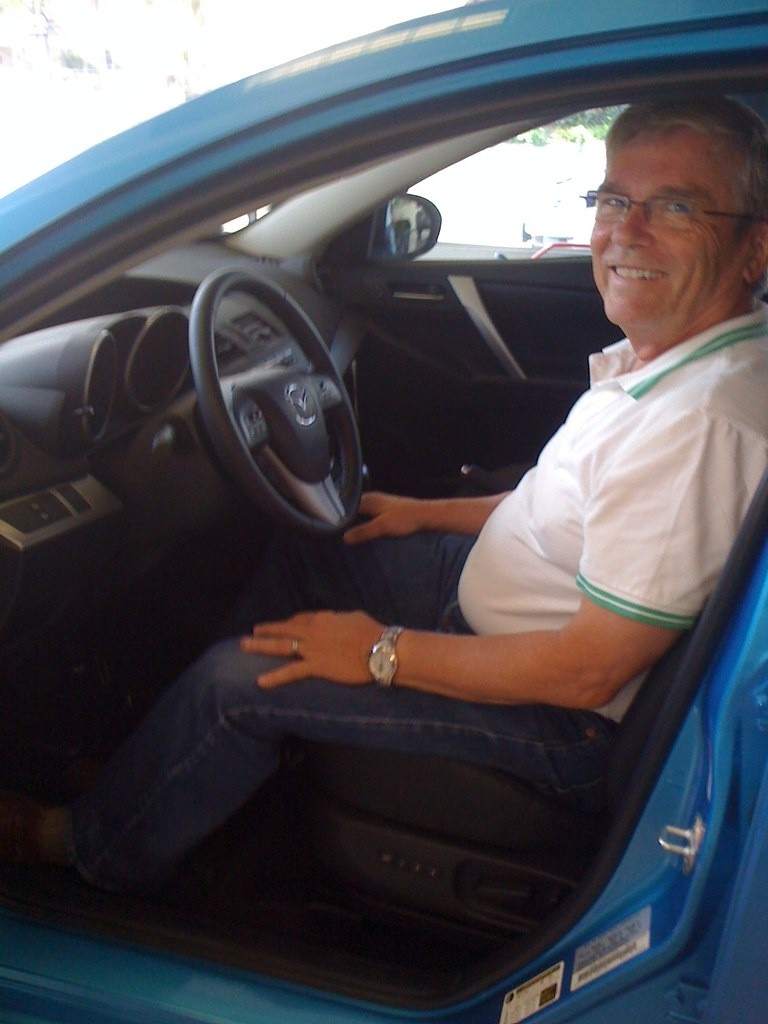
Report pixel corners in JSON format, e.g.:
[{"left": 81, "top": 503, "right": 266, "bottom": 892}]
[{"left": 292, "top": 640, "right": 298, "bottom": 654}]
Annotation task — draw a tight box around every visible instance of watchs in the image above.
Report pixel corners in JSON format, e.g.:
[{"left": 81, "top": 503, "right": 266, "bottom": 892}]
[{"left": 367, "top": 625, "right": 405, "bottom": 687}]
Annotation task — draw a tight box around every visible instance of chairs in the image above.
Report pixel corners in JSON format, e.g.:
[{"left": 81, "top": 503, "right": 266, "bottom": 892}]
[{"left": 296, "top": 468, "right": 768, "bottom": 979}]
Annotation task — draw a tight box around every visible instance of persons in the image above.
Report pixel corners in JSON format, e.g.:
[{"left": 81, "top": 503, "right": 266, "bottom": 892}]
[{"left": 1, "top": 95, "right": 768, "bottom": 888}]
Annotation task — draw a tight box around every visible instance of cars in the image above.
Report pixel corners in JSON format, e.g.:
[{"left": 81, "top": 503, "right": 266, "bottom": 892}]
[{"left": 0, "top": 1, "right": 767, "bottom": 1023}]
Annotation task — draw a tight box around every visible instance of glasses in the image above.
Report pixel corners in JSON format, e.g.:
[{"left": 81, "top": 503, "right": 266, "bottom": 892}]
[{"left": 578, "top": 189, "right": 768, "bottom": 231}]
